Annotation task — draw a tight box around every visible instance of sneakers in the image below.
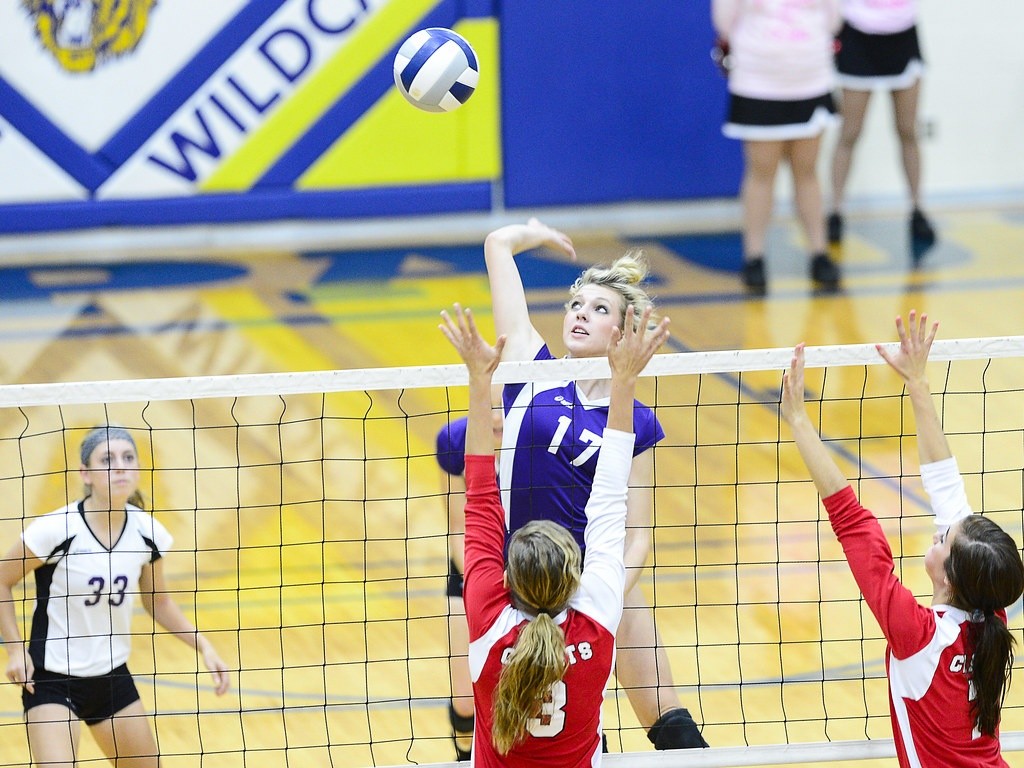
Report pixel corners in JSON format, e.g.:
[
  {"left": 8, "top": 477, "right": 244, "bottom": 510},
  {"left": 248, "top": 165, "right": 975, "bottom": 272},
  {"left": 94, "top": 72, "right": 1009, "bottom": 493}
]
[
  {"left": 823, "top": 215, "right": 845, "bottom": 244},
  {"left": 908, "top": 211, "right": 935, "bottom": 260},
  {"left": 809, "top": 251, "right": 848, "bottom": 290},
  {"left": 741, "top": 256, "right": 766, "bottom": 293}
]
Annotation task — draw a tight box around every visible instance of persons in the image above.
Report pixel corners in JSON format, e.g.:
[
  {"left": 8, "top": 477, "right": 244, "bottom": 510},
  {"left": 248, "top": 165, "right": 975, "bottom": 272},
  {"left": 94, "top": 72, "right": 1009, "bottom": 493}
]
[
  {"left": 484, "top": 214, "right": 715, "bottom": 749},
  {"left": 0, "top": 422, "right": 230, "bottom": 767},
  {"left": 438, "top": 297, "right": 672, "bottom": 768},
  {"left": 436, "top": 379, "right": 507, "bottom": 766},
  {"left": 827, "top": 0, "right": 935, "bottom": 257},
  {"left": 779, "top": 307, "right": 1024, "bottom": 768},
  {"left": 709, "top": 0, "right": 849, "bottom": 300}
]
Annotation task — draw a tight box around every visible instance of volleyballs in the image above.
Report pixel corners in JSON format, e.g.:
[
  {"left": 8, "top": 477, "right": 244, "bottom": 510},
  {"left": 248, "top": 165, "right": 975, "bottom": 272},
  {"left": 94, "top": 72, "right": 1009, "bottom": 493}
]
[{"left": 393, "top": 26, "right": 480, "bottom": 116}]
